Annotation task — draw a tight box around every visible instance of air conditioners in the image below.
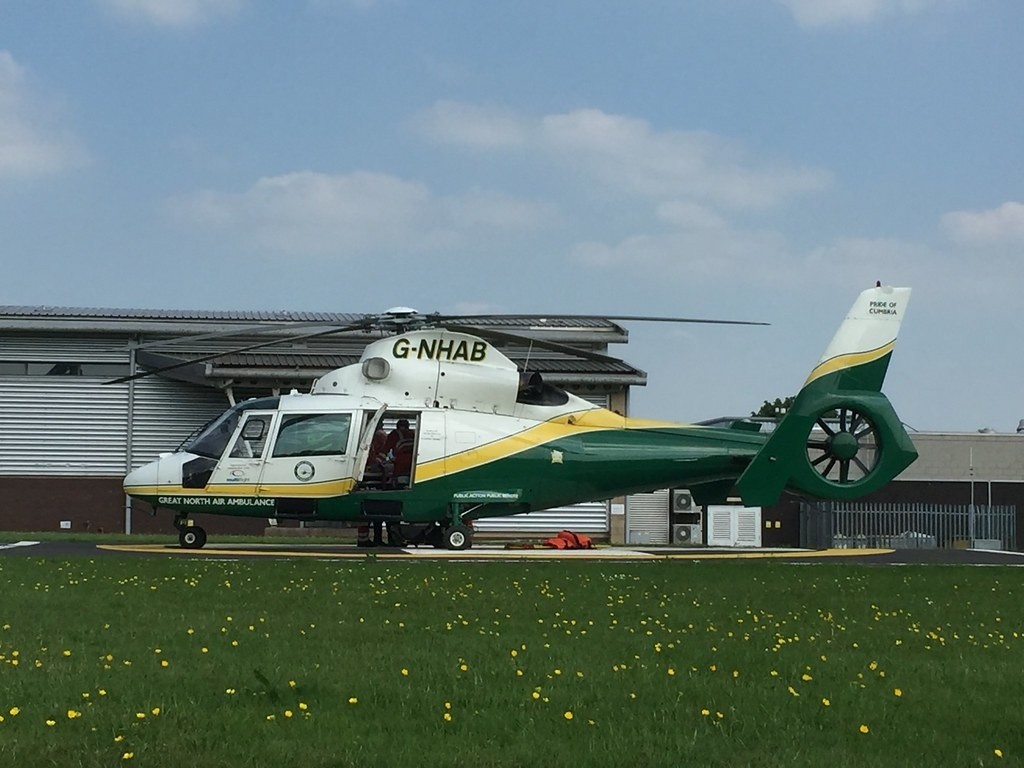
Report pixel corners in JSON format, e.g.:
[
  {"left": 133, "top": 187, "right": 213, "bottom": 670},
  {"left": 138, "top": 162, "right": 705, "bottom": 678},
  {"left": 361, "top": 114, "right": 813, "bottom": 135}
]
[
  {"left": 671, "top": 524, "right": 702, "bottom": 546},
  {"left": 671, "top": 489, "right": 701, "bottom": 513}
]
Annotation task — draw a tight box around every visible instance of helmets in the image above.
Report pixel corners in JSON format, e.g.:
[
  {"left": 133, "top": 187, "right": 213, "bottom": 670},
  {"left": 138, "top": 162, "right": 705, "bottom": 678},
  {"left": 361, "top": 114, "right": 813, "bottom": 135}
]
[
  {"left": 396, "top": 419, "right": 409, "bottom": 428},
  {"left": 366, "top": 412, "right": 384, "bottom": 427}
]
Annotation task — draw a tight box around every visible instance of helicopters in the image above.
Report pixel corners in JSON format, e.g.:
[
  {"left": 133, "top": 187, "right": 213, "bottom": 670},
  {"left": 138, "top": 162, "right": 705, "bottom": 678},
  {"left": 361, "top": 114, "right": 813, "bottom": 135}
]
[{"left": 102, "top": 280, "right": 920, "bottom": 550}]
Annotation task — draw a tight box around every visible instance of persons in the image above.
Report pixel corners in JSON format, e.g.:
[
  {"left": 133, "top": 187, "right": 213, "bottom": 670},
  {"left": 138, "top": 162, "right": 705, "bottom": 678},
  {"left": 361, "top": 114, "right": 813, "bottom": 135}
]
[
  {"left": 356, "top": 430, "right": 390, "bottom": 548},
  {"left": 375, "top": 417, "right": 415, "bottom": 486}
]
